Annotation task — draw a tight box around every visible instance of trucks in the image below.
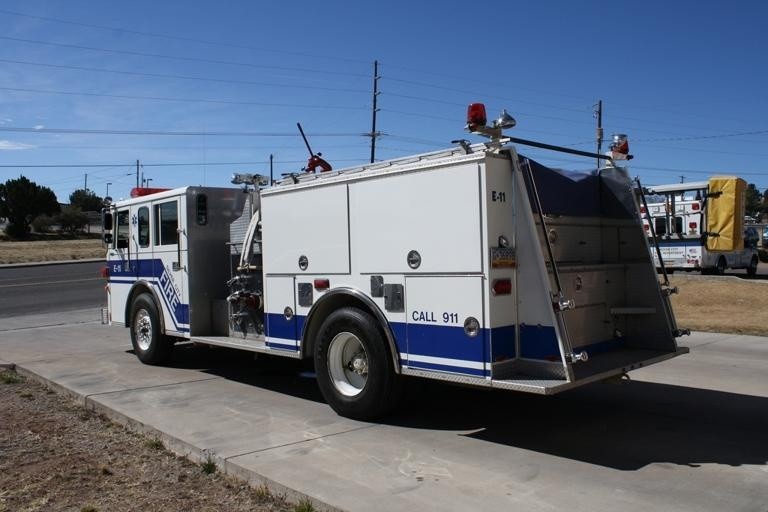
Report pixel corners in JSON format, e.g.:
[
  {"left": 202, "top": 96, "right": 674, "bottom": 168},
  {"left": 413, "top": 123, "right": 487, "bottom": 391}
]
[{"left": 636, "top": 178, "right": 760, "bottom": 279}]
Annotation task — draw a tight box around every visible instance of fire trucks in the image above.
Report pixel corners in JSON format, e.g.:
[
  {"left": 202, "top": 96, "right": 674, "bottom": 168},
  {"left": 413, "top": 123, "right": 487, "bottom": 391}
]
[{"left": 99, "top": 100, "right": 692, "bottom": 424}]
